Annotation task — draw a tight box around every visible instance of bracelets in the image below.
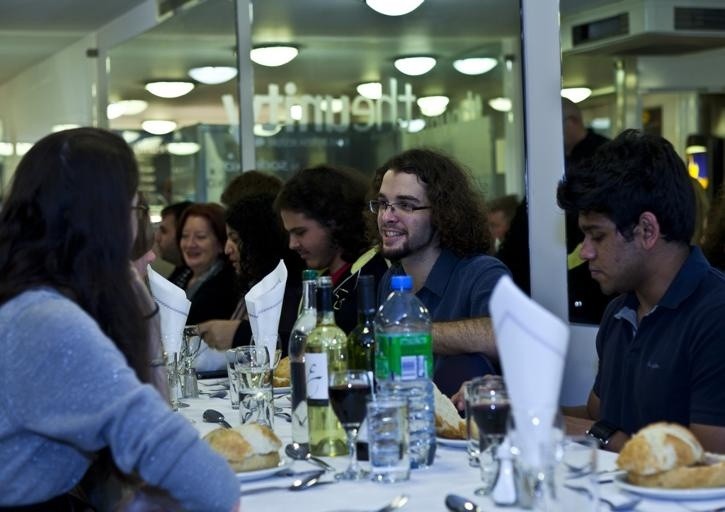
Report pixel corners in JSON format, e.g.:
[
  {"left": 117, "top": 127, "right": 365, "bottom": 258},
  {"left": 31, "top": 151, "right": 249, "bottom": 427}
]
[{"left": 583, "top": 416, "right": 624, "bottom": 450}]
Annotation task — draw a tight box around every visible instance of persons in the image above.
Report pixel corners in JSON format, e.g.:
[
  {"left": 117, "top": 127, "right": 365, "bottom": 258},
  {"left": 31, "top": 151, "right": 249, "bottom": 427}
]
[
  {"left": 550, "top": 126, "right": 724, "bottom": 457},
  {"left": 363, "top": 144, "right": 523, "bottom": 431},
  {"left": 483, "top": 193, "right": 520, "bottom": 245},
  {"left": 500, "top": 93, "right": 615, "bottom": 326},
  {"left": 0, "top": 126, "right": 244, "bottom": 511},
  {"left": 130, "top": 169, "right": 307, "bottom": 379},
  {"left": 186, "top": 163, "right": 388, "bottom": 401}
]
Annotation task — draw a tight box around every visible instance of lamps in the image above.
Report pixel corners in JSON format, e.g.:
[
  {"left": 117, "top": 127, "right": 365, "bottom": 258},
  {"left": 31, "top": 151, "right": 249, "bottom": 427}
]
[
  {"left": 491, "top": 97, "right": 513, "bottom": 110},
  {"left": 452, "top": 57, "right": 497, "bottom": 76},
  {"left": 141, "top": 121, "right": 176, "bottom": 136},
  {"left": 251, "top": 45, "right": 300, "bottom": 69},
  {"left": 559, "top": 87, "right": 591, "bottom": 104},
  {"left": 189, "top": 64, "right": 238, "bottom": 85},
  {"left": 106, "top": 100, "right": 148, "bottom": 120},
  {"left": 369, "top": 1, "right": 423, "bottom": 17},
  {"left": 397, "top": 95, "right": 450, "bottom": 134},
  {"left": 167, "top": 141, "right": 201, "bottom": 155},
  {"left": 249, "top": 83, "right": 383, "bottom": 139},
  {"left": 395, "top": 56, "right": 437, "bottom": 76},
  {"left": 146, "top": 81, "right": 193, "bottom": 100}
]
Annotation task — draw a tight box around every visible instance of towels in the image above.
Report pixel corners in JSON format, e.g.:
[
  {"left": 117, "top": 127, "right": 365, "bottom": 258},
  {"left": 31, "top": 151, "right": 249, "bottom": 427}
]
[
  {"left": 484, "top": 272, "right": 572, "bottom": 480},
  {"left": 240, "top": 256, "right": 290, "bottom": 366},
  {"left": 144, "top": 259, "right": 194, "bottom": 363}
]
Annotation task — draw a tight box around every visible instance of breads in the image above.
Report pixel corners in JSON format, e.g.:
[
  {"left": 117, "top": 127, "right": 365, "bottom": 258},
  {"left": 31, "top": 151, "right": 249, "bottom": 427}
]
[
  {"left": 615, "top": 420, "right": 724, "bottom": 489},
  {"left": 202, "top": 421, "right": 284, "bottom": 472},
  {"left": 431, "top": 382, "right": 478, "bottom": 440},
  {"left": 272, "top": 355, "right": 292, "bottom": 387}
]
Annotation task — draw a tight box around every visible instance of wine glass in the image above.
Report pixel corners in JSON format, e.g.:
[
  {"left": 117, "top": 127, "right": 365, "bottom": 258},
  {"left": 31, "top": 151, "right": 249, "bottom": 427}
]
[{"left": 178, "top": 323, "right": 203, "bottom": 366}]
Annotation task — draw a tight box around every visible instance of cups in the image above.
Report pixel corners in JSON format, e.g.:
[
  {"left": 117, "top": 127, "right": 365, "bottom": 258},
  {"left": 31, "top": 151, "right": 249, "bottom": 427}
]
[
  {"left": 460, "top": 375, "right": 512, "bottom": 483},
  {"left": 223, "top": 344, "right": 280, "bottom": 429},
  {"left": 541, "top": 434, "right": 600, "bottom": 511}
]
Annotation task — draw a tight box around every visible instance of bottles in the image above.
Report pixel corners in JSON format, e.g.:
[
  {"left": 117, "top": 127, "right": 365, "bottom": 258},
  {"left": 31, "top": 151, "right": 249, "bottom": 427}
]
[
  {"left": 287, "top": 262, "right": 438, "bottom": 485},
  {"left": 165, "top": 365, "right": 199, "bottom": 411},
  {"left": 488, "top": 445, "right": 520, "bottom": 507}
]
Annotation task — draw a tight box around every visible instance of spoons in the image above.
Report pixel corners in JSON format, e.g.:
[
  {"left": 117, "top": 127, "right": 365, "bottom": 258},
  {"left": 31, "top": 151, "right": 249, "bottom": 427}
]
[
  {"left": 241, "top": 470, "right": 325, "bottom": 498},
  {"left": 202, "top": 407, "right": 234, "bottom": 429},
  {"left": 284, "top": 441, "right": 334, "bottom": 473},
  {"left": 567, "top": 484, "right": 641, "bottom": 512},
  {"left": 442, "top": 493, "right": 482, "bottom": 511}
]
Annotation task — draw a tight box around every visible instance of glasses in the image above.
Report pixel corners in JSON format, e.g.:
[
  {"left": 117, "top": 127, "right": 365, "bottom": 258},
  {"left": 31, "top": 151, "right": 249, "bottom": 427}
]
[
  {"left": 368, "top": 199, "right": 435, "bottom": 215},
  {"left": 128, "top": 203, "right": 149, "bottom": 220}
]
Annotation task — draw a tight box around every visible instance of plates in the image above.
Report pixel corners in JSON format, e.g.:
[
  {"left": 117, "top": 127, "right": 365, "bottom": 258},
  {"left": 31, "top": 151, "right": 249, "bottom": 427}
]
[
  {"left": 437, "top": 435, "right": 471, "bottom": 451},
  {"left": 232, "top": 460, "right": 292, "bottom": 484},
  {"left": 267, "top": 385, "right": 291, "bottom": 394},
  {"left": 614, "top": 472, "right": 725, "bottom": 500}
]
